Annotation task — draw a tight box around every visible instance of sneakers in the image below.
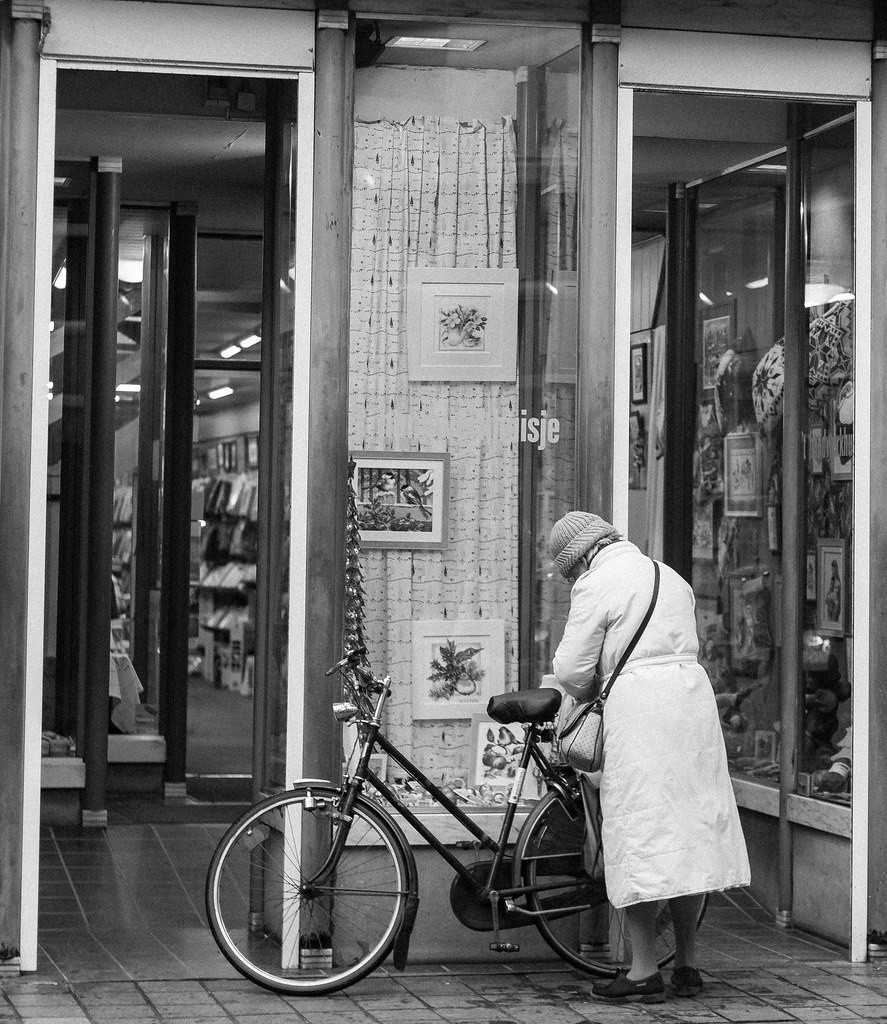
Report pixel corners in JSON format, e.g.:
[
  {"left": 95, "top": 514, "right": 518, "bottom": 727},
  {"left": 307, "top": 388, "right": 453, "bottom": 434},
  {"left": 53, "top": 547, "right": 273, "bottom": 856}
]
[
  {"left": 671, "top": 967, "right": 703, "bottom": 997},
  {"left": 590, "top": 967, "right": 666, "bottom": 1003}
]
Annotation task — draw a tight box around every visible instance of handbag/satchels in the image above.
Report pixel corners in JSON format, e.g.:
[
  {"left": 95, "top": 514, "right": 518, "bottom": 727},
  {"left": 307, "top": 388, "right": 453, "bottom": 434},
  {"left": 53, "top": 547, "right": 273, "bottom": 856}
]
[{"left": 559, "top": 698, "right": 604, "bottom": 772}]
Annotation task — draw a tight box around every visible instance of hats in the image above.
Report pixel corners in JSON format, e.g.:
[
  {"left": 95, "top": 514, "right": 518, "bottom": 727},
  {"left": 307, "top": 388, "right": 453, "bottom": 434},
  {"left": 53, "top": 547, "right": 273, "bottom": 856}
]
[{"left": 549, "top": 511, "right": 616, "bottom": 578}]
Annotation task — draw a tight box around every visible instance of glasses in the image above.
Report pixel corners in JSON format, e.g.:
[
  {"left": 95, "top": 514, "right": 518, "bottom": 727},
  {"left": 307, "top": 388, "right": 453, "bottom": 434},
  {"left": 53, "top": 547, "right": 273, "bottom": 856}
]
[{"left": 567, "top": 561, "right": 579, "bottom": 582}]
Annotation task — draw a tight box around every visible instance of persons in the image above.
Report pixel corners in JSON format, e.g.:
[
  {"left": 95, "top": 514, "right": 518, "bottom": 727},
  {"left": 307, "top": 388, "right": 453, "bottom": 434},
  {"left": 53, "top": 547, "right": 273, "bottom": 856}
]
[
  {"left": 548, "top": 511, "right": 752, "bottom": 1003},
  {"left": 733, "top": 458, "right": 750, "bottom": 490},
  {"left": 824, "top": 561, "right": 840, "bottom": 620}
]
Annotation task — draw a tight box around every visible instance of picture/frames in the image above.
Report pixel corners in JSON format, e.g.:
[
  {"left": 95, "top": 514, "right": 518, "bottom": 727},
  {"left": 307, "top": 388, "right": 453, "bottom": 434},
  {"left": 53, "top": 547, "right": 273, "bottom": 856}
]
[
  {"left": 407, "top": 267, "right": 518, "bottom": 382},
  {"left": 766, "top": 503, "right": 782, "bottom": 553},
  {"left": 691, "top": 491, "right": 719, "bottom": 568},
  {"left": 698, "top": 298, "right": 737, "bottom": 401},
  {"left": 545, "top": 270, "right": 577, "bottom": 383},
  {"left": 810, "top": 424, "right": 825, "bottom": 477},
  {"left": 816, "top": 539, "right": 852, "bottom": 638},
  {"left": 805, "top": 550, "right": 815, "bottom": 601},
  {"left": 468, "top": 717, "right": 525, "bottom": 790},
  {"left": 727, "top": 573, "right": 757, "bottom": 677},
  {"left": 724, "top": 432, "right": 762, "bottom": 518},
  {"left": 830, "top": 399, "right": 852, "bottom": 480},
  {"left": 630, "top": 343, "right": 647, "bottom": 404},
  {"left": 412, "top": 619, "right": 505, "bottom": 719},
  {"left": 366, "top": 754, "right": 388, "bottom": 795},
  {"left": 348, "top": 450, "right": 450, "bottom": 550},
  {"left": 629, "top": 411, "right": 642, "bottom": 441}
]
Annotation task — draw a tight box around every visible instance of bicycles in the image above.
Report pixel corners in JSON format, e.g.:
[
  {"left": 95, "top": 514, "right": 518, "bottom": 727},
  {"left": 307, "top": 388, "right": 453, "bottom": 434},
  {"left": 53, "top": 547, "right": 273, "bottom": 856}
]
[{"left": 205, "top": 648, "right": 710, "bottom": 997}]
